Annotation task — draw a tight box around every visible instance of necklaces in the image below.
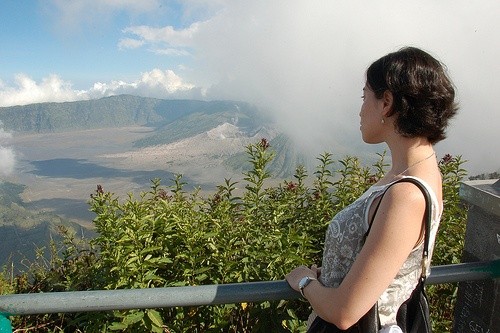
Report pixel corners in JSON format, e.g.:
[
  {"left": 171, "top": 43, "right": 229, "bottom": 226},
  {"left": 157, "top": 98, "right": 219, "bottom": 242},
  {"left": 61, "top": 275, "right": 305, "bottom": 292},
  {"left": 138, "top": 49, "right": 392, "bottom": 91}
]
[{"left": 384, "top": 151, "right": 435, "bottom": 184}]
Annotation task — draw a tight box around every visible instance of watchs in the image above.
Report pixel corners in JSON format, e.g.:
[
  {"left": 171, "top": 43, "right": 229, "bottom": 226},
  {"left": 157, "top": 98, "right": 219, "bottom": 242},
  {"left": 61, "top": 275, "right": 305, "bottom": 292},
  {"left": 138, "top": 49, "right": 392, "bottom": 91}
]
[{"left": 299, "top": 277, "right": 318, "bottom": 299}]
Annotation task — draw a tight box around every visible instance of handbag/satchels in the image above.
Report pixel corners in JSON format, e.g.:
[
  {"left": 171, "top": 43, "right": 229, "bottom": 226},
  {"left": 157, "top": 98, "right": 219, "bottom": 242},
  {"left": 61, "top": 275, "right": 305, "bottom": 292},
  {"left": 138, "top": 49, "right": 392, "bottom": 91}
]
[{"left": 304, "top": 178, "right": 432, "bottom": 333}]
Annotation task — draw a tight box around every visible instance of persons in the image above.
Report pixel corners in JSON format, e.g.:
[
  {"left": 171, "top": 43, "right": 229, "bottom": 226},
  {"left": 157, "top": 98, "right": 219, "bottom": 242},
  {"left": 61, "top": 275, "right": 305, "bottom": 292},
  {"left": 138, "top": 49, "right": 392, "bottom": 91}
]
[{"left": 284, "top": 46, "right": 460, "bottom": 333}]
